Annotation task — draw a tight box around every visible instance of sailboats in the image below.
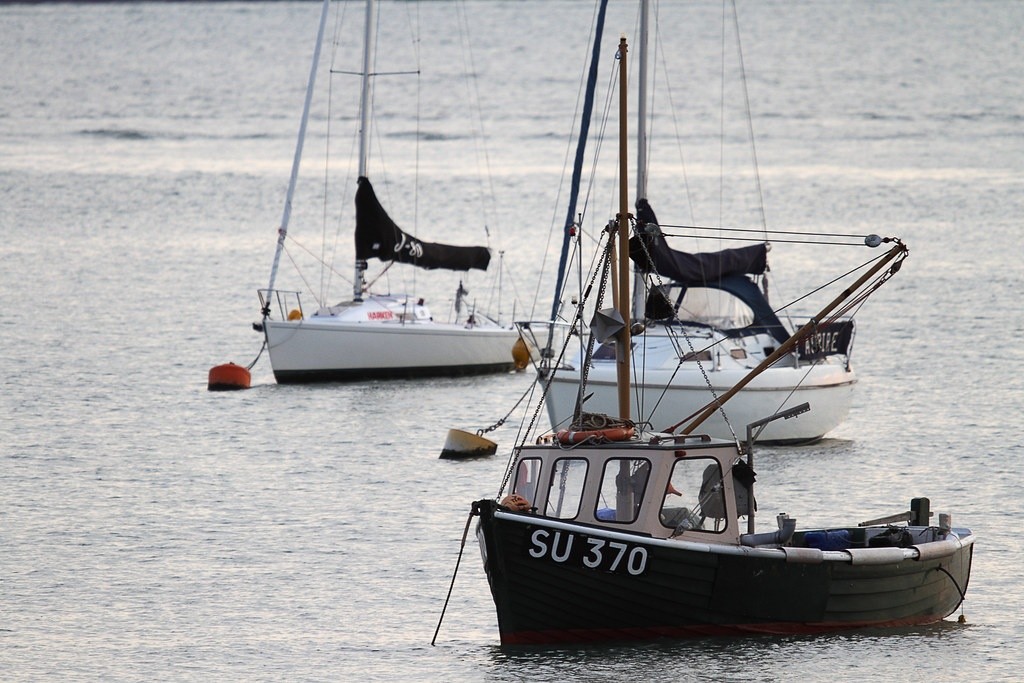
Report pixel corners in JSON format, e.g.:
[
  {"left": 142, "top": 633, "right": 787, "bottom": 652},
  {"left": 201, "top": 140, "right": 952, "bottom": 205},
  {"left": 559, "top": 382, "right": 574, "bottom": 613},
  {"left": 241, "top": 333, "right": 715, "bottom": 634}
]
[
  {"left": 465, "top": 32, "right": 978, "bottom": 648},
  {"left": 250, "top": 0, "right": 562, "bottom": 384},
  {"left": 512, "top": 2, "right": 864, "bottom": 446}
]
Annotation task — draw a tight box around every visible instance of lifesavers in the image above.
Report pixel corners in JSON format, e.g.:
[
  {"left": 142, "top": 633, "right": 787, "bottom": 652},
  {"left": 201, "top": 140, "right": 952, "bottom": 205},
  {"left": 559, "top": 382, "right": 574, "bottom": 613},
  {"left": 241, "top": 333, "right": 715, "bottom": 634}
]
[{"left": 558, "top": 427, "right": 634, "bottom": 444}]
